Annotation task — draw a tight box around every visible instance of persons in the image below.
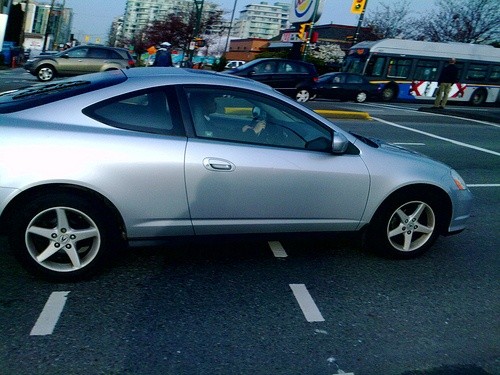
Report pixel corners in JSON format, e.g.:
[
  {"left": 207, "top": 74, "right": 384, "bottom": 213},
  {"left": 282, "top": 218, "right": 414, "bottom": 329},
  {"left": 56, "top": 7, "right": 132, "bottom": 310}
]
[
  {"left": 433, "top": 57, "right": 459, "bottom": 108},
  {"left": 148, "top": 41, "right": 173, "bottom": 109},
  {"left": 202, "top": 91, "right": 267, "bottom": 141},
  {"left": 184, "top": 56, "right": 194, "bottom": 68}
]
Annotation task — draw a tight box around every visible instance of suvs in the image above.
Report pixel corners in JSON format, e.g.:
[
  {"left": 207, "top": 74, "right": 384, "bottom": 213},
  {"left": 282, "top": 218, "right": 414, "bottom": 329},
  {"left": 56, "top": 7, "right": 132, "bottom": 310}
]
[
  {"left": 215, "top": 59, "right": 321, "bottom": 104},
  {"left": 24, "top": 44, "right": 136, "bottom": 82}
]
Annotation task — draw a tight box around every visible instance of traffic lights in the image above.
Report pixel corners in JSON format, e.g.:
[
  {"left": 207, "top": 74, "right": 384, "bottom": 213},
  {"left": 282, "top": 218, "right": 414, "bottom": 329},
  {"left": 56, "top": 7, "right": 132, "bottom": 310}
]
[{"left": 296, "top": 24, "right": 305, "bottom": 39}]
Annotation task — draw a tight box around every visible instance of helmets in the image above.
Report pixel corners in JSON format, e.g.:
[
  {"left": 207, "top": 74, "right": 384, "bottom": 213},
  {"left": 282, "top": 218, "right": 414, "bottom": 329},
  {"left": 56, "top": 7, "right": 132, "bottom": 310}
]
[{"left": 160, "top": 42, "right": 172, "bottom": 46}]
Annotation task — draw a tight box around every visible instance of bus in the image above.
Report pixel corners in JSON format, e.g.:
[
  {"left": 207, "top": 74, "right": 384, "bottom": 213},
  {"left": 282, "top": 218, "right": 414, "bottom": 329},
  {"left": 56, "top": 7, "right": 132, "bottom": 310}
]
[{"left": 338, "top": 37, "right": 500, "bottom": 106}]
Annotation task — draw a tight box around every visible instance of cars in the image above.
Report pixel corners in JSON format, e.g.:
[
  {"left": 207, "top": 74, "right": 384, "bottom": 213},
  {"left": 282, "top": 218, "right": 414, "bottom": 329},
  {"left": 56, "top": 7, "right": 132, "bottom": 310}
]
[
  {"left": 310, "top": 72, "right": 375, "bottom": 103},
  {"left": 0, "top": 65, "right": 477, "bottom": 285}
]
